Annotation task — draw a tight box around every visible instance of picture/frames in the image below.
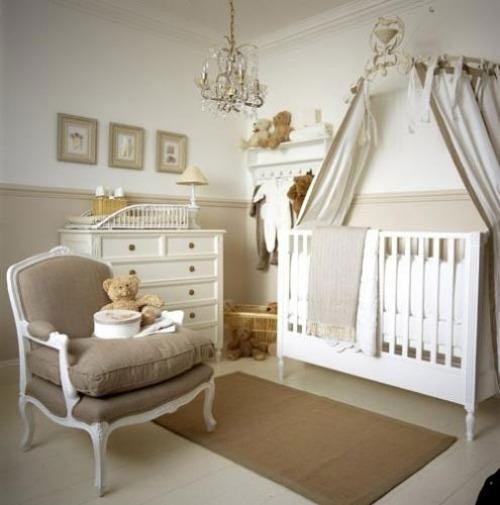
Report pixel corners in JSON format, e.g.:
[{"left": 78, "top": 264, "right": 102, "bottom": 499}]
[
  {"left": 109, "top": 122, "right": 144, "bottom": 170},
  {"left": 57, "top": 112, "right": 97, "bottom": 165},
  {"left": 155, "top": 130, "right": 188, "bottom": 174}
]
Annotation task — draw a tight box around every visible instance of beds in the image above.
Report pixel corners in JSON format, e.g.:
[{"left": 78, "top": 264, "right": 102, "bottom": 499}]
[{"left": 276, "top": 226, "right": 499, "bottom": 443}]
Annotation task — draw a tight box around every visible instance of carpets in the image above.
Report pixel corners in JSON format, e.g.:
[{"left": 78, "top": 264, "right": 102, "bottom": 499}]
[{"left": 150, "top": 371, "right": 458, "bottom": 505}]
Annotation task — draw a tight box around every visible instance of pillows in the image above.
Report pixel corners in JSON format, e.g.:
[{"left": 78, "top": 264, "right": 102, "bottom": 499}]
[{"left": 26, "top": 321, "right": 214, "bottom": 396}]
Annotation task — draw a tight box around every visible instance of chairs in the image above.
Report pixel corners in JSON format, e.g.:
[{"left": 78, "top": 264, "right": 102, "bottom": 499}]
[{"left": 6, "top": 245, "right": 216, "bottom": 498}]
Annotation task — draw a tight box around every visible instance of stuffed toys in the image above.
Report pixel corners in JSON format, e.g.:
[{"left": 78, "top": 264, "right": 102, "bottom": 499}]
[
  {"left": 265, "top": 303, "right": 277, "bottom": 343},
  {"left": 227, "top": 326, "right": 268, "bottom": 361},
  {"left": 258, "top": 111, "right": 293, "bottom": 149},
  {"left": 100, "top": 275, "right": 165, "bottom": 327},
  {"left": 287, "top": 175, "right": 313, "bottom": 215},
  {"left": 241, "top": 119, "right": 272, "bottom": 150}
]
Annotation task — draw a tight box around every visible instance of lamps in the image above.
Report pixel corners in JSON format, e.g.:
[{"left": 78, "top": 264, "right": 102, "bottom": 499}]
[
  {"left": 194, "top": 0, "right": 268, "bottom": 113},
  {"left": 176, "top": 165, "right": 211, "bottom": 229}
]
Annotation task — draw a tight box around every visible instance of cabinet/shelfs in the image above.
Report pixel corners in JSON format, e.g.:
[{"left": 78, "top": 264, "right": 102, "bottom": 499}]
[
  {"left": 246, "top": 135, "right": 332, "bottom": 180},
  {"left": 57, "top": 228, "right": 228, "bottom": 363}
]
